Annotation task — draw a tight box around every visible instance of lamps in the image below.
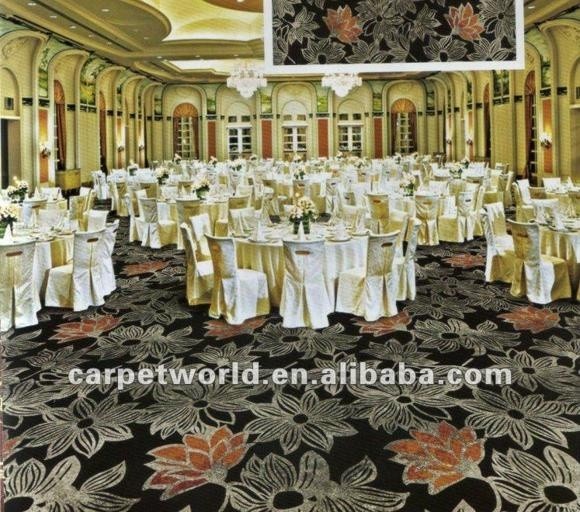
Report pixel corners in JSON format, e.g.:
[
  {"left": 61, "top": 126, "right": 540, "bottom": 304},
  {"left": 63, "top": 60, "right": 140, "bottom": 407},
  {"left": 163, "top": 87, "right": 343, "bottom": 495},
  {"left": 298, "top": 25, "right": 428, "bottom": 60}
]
[
  {"left": 540, "top": 134, "right": 552, "bottom": 149},
  {"left": 118, "top": 142, "right": 125, "bottom": 153},
  {"left": 465, "top": 132, "right": 473, "bottom": 147},
  {"left": 319, "top": 71, "right": 364, "bottom": 100},
  {"left": 446, "top": 134, "right": 454, "bottom": 146},
  {"left": 138, "top": 142, "right": 145, "bottom": 152},
  {"left": 38, "top": 140, "right": 53, "bottom": 160},
  {"left": 224, "top": 58, "right": 269, "bottom": 101}
]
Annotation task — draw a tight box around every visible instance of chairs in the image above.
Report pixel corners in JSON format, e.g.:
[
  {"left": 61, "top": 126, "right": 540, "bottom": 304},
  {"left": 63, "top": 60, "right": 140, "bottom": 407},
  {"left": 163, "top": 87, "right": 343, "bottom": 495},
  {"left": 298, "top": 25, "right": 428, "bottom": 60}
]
[{"left": 0, "top": 148, "right": 580, "bottom": 337}]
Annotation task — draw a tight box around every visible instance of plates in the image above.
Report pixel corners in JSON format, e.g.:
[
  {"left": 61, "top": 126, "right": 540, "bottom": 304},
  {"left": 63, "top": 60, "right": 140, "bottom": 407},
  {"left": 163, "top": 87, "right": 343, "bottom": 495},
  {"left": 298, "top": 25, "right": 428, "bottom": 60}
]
[
  {"left": 530, "top": 213, "right": 579, "bottom": 234},
  {"left": 1, "top": 212, "right": 79, "bottom": 245},
  {"left": 226, "top": 212, "right": 367, "bottom": 245}
]
